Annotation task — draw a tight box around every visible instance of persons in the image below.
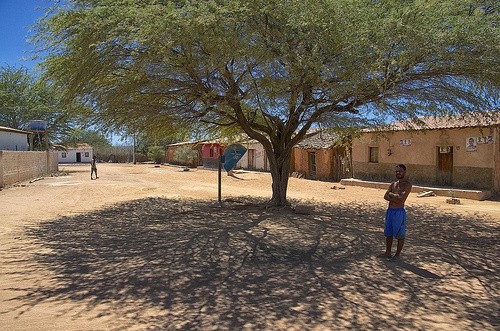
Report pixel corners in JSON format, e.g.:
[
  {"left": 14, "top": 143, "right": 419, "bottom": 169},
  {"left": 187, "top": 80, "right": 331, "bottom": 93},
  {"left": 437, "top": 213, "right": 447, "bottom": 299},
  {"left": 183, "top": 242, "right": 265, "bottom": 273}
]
[
  {"left": 466, "top": 138, "right": 476, "bottom": 152},
  {"left": 377, "top": 164, "right": 412, "bottom": 260},
  {"left": 91, "top": 156, "right": 99, "bottom": 180},
  {"left": 477, "top": 135, "right": 494, "bottom": 145}
]
